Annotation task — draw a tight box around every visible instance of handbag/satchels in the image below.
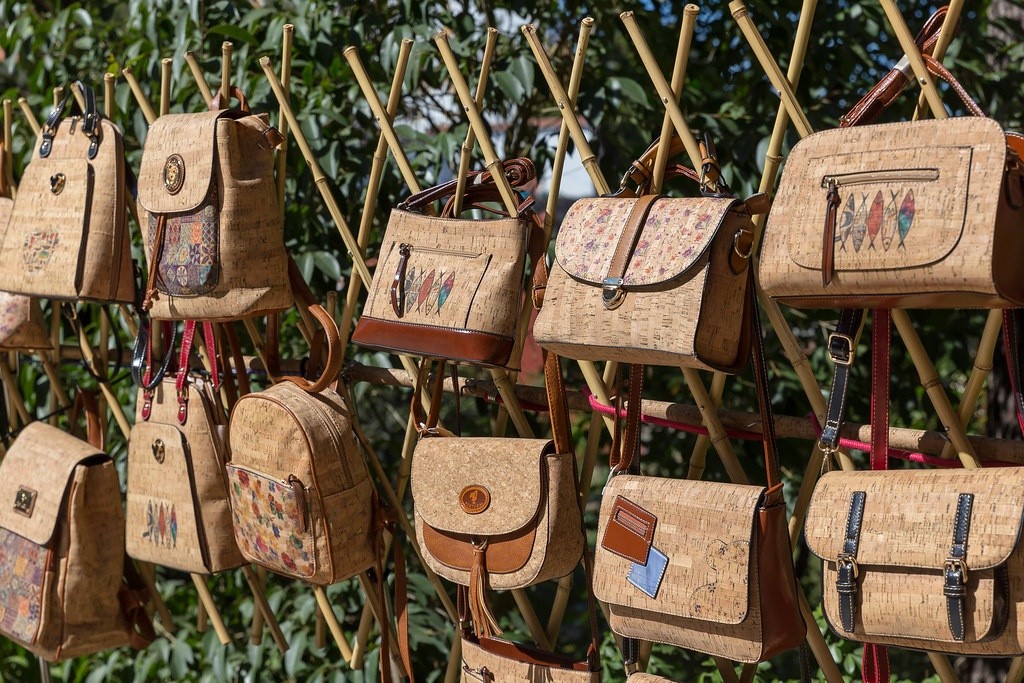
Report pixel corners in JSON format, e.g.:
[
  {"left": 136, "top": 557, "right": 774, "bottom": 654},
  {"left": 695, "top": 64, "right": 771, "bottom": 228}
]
[
  {"left": 124, "top": 320, "right": 252, "bottom": 576},
  {"left": 0, "top": 81, "right": 134, "bottom": 305},
  {"left": 803, "top": 307, "right": 1024, "bottom": 658},
  {"left": 459, "top": 632, "right": 602, "bottom": 683},
  {"left": 592, "top": 464, "right": 807, "bottom": 664},
  {"left": 756, "top": 7, "right": 1024, "bottom": 309},
  {"left": 533, "top": 131, "right": 770, "bottom": 375},
  {"left": 0, "top": 389, "right": 157, "bottom": 664},
  {"left": 351, "top": 157, "right": 536, "bottom": 368},
  {"left": 0, "top": 143, "right": 57, "bottom": 351},
  {"left": 136, "top": 85, "right": 295, "bottom": 320},
  {"left": 226, "top": 379, "right": 386, "bottom": 587},
  {"left": 411, "top": 436, "right": 584, "bottom": 639}
]
